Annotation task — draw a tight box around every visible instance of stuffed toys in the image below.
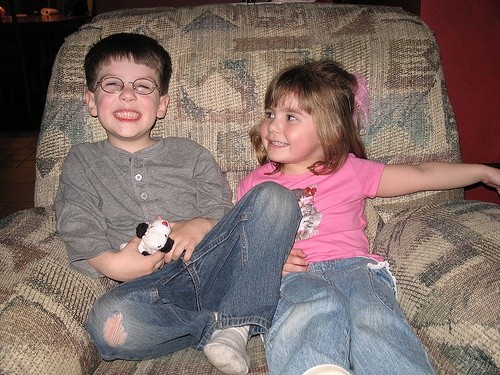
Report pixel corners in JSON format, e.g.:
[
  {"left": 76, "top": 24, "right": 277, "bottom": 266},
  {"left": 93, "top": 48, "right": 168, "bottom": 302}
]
[{"left": 121, "top": 220, "right": 175, "bottom": 256}]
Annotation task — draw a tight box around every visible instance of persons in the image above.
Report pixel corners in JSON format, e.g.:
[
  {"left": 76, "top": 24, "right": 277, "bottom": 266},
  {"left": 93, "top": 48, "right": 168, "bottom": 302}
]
[
  {"left": 235, "top": 57, "right": 500, "bottom": 375},
  {"left": 55, "top": 33, "right": 302, "bottom": 375}
]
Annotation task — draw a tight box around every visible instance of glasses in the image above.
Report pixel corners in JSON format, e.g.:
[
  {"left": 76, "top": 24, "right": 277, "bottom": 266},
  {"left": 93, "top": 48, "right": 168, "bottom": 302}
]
[{"left": 93, "top": 77, "right": 161, "bottom": 95}]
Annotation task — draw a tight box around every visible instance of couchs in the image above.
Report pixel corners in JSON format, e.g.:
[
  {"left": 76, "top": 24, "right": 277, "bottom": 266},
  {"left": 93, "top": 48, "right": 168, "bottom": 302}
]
[{"left": 0, "top": 3, "right": 500, "bottom": 375}]
